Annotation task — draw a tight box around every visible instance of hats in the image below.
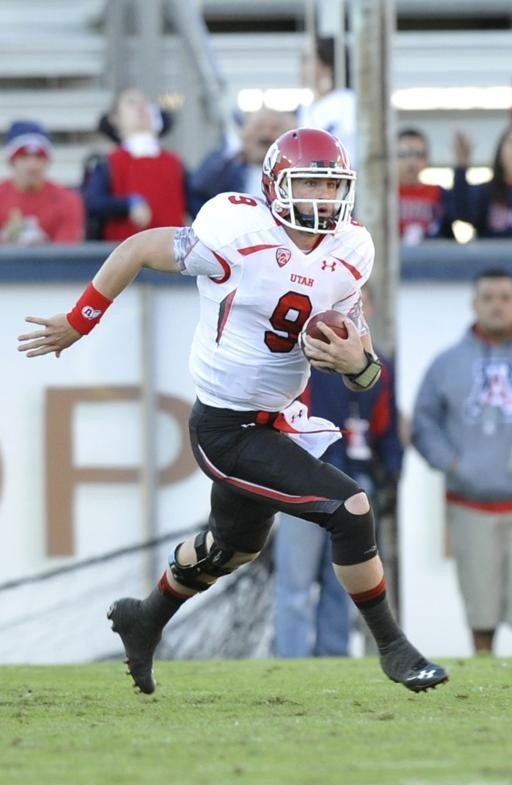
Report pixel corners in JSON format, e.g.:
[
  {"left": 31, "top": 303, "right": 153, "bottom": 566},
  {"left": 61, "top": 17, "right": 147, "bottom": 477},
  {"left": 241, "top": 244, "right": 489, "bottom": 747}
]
[{"left": 4, "top": 119, "right": 53, "bottom": 167}]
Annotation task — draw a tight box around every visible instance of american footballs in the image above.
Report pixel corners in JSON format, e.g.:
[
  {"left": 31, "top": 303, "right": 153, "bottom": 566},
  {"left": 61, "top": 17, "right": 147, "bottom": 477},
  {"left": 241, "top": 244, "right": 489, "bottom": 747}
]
[{"left": 297, "top": 311, "right": 350, "bottom": 376}]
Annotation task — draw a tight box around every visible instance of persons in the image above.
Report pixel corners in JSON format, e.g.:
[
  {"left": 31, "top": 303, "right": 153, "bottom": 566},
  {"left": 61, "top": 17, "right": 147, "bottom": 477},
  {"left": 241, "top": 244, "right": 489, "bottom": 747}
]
[
  {"left": 272, "top": 279, "right": 413, "bottom": 657},
  {"left": 19, "top": 128, "right": 450, "bottom": 692},
  {"left": 410, "top": 270, "right": 512, "bottom": 656},
  {"left": 1, "top": 35, "right": 512, "bottom": 245}
]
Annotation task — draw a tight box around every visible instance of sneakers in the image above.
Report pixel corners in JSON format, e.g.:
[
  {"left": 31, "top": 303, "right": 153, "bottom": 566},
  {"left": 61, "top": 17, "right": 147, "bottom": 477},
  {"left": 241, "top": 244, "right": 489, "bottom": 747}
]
[
  {"left": 106, "top": 597, "right": 156, "bottom": 694},
  {"left": 379, "top": 645, "right": 449, "bottom": 692}
]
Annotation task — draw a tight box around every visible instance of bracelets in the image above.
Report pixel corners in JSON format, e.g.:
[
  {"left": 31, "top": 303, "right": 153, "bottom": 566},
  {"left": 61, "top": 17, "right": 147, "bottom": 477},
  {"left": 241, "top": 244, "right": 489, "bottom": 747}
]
[
  {"left": 66, "top": 280, "right": 113, "bottom": 336},
  {"left": 346, "top": 350, "right": 383, "bottom": 392}
]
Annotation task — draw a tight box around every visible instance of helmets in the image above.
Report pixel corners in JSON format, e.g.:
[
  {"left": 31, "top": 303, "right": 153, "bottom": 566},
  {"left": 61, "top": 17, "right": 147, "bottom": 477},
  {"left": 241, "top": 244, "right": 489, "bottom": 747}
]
[{"left": 262, "top": 128, "right": 355, "bottom": 238}]
